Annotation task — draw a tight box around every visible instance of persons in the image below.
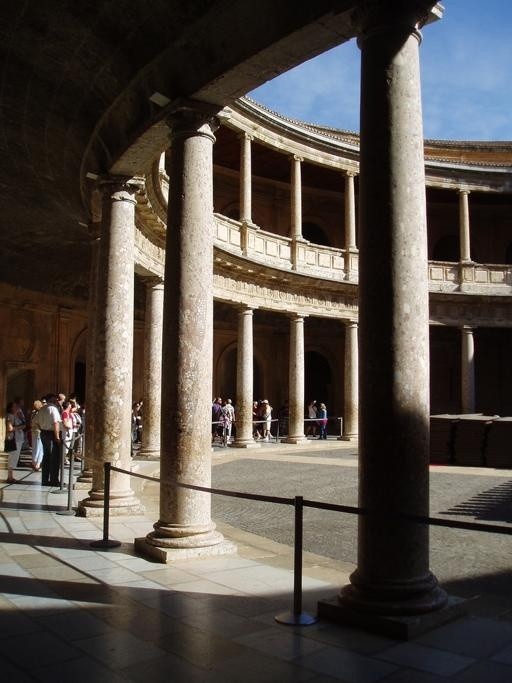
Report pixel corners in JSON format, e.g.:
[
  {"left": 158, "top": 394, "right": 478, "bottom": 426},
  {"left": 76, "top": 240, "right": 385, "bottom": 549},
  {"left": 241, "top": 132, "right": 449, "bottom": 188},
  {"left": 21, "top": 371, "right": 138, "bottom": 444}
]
[
  {"left": 5, "top": 393, "right": 86, "bottom": 487},
  {"left": 212, "top": 397, "right": 236, "bottom": 440},
  {"left": 318, "top": 403, "right": 328, "bottom": 440},
  {"left": 304, "top": 400, "right": 318, "bottom": 437},
  {"left": 132, "top": 400, "right": 144, "bottom": 442},
  {"left": 254, "top": 397, "right": 289, "bottom": 440}
]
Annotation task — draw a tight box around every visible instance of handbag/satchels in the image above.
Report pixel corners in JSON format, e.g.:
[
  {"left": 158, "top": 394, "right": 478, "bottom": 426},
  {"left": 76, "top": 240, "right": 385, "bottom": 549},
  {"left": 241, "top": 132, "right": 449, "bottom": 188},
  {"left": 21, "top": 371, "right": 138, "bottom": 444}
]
[{"left": 4, "top": 436, "right": 17, "bottom": 453}]
